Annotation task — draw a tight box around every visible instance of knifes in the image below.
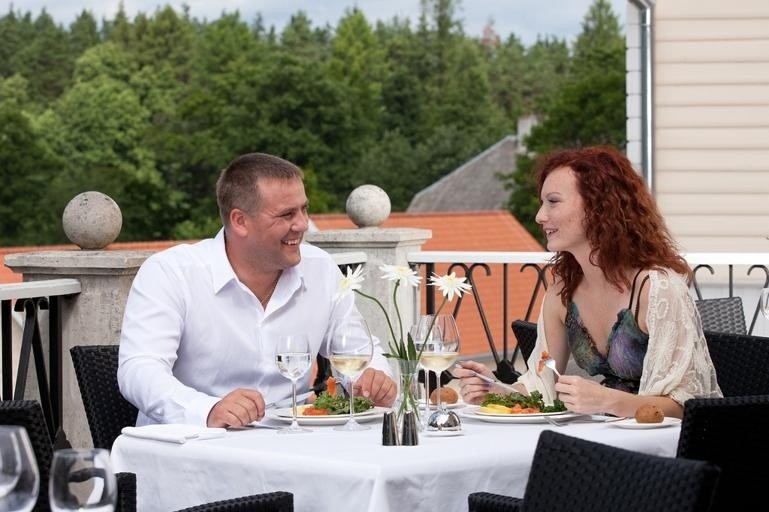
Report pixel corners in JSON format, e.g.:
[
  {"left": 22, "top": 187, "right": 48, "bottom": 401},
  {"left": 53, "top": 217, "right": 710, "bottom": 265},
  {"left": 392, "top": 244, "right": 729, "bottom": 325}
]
[
  {"left": 262, "top": 391, "right": 314, "bottom": 414},
  {"left": 455, "top": 362, "right": 528, "bottom": 399}
]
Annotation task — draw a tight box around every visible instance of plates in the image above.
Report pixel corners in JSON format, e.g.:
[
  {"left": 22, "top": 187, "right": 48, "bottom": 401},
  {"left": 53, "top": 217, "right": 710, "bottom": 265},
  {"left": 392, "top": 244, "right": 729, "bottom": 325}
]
[
  {"left": 458, "top": 407, "right": 583, "bottom": 423},
  {"left": 417, "top": 400, "right": 465, "bottom": 410},
  {"left": 263, "top": 406, "right": 393, "bottom": 424},
  {"left": 612, "top": 418, "right": 681, "bottom": 430}
]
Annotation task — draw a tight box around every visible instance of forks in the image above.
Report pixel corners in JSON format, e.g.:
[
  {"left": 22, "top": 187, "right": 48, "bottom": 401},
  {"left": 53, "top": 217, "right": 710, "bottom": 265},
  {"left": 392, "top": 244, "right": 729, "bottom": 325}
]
[{"left": 540, "top": 348, "right": 563, "bottom": 377}]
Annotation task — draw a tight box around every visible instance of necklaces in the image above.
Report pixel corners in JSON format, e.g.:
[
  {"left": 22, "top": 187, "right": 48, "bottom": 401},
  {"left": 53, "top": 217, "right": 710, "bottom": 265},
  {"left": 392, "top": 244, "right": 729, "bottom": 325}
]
[{"left": 260, "top": 279, "right": 278, "bottom": 304}]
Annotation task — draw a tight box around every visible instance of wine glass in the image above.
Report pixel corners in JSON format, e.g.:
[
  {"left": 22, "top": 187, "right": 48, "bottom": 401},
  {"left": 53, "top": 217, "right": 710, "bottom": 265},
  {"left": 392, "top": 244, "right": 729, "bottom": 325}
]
[
  {"left": 0, "top": 426, "right": 40, "bottom": 512},
  {"left": 48, "top": 448, "right": 119, "bottom": 512},
  {"left": 329, "top": 317, "right": 373, "bottom": 430},
  {"left": 411, "top": 312, "right": 464, "bottom": 431},
  {"left": 273, "top": 335, "right": 315, "bottom": 435}
]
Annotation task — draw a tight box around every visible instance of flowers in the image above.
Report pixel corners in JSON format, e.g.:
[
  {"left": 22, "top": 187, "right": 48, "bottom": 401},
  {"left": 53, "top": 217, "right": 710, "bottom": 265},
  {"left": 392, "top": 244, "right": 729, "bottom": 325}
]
[{"left": 333, "top": 264, "right": 471, "bottom": 421}]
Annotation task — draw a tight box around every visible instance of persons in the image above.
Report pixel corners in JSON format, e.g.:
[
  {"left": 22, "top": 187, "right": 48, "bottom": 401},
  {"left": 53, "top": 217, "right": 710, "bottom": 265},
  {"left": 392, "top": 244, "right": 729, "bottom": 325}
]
[
  {"left": 452, "top": 139, "right": 726, "bottom": 420},
  {"left": 115, "top": 152, "right": 400, "bottom": 430}
]
